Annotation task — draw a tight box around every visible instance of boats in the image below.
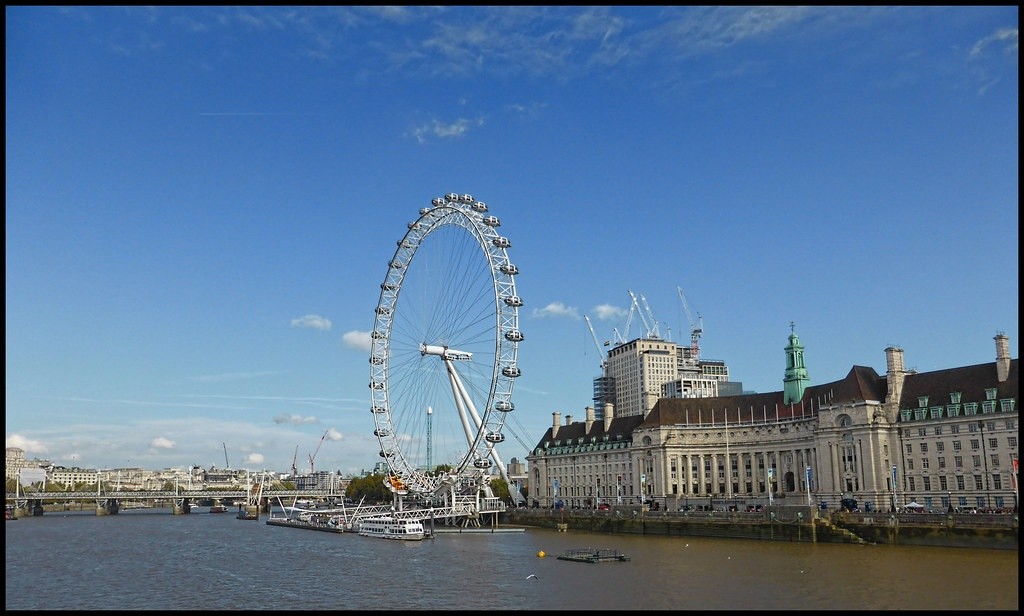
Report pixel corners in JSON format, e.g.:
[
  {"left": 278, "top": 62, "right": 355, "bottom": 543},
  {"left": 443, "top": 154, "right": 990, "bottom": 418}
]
[
  {"left": 209, "top": 505, "right": 229, "bottom": 513},
  {"left": 357, "top": 517, "right": 425, "bottom": 540},
  {"left": 237, "top": 504, "right": 262, "bottom": 521},
  {"left": 557, "top": 547, "right": 633, "bottom": 564}
]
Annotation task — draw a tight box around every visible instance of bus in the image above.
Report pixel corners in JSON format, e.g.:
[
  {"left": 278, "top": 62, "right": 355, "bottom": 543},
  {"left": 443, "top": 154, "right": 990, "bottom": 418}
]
[{"left": 958, "top": 506, "right": 978, "bottom": 513}]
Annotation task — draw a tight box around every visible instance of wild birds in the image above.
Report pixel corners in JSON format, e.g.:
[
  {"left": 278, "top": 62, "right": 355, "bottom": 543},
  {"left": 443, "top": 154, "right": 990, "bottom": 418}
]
[{"left": 526, "top": 574, "right": 538, "bottom": 580}]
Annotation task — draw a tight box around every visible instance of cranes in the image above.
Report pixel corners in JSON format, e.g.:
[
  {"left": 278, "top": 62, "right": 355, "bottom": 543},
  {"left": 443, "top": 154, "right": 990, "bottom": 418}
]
[
  {"left": 309, "top": 430, "right": 330, "bottom": 473},
  {"left": 582, "top": 284, "right": 703, "bottom": 371},
  {"left": 223, "top": 442, "right": 229, "bottom": 469},
  {"left": 291, "top": 445, "right": 299, "bottom": 476}
]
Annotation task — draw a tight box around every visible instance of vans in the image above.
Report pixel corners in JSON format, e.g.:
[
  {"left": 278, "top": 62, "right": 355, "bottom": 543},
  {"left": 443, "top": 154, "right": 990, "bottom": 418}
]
[{"left": 550, "top": 501, "right": 563, "bottom": 509}]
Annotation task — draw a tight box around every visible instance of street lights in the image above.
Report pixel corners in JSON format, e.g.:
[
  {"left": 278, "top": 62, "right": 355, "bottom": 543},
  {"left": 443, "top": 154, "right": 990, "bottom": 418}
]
[
  {"left": 947, "top": 491, "right": 954, "bottom": 513},
  {"left": 840, "top": 493, "right": 846, "bottom": 512},
  {"left": 664, "top": 495, "right": 667, "bottom": 512},
  {"left": 532, "top": 497, "right": 535, "bottom": 509},
  {"left": 685, "top": 495, "right": 689, "bottom": 511},
  {"left": 890, "top": 492, "right": 896, "bottom": 512},
  {"left": 708, "top": 494, "right": 714, "bottom": 511},
  {"left": 734, "top": 494, "right": 737, "bottom": 512},
  {"left": 571, "top": 495, "right": 575, "bottom": 509},
  {"left": 514, "top": 497, "right": 517, "bottom": 508}
]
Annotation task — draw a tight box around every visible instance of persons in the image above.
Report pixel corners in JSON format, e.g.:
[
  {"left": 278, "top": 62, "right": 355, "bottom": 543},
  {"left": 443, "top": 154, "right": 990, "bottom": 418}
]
[{"left": 836, "top": 506, "right": 1002, "bottom": 514}]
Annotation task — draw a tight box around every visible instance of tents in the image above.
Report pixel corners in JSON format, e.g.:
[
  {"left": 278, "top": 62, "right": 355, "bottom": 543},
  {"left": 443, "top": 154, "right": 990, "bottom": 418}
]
[{"left": 904, "top": 502, "right": 924, "bottom": 513}]
